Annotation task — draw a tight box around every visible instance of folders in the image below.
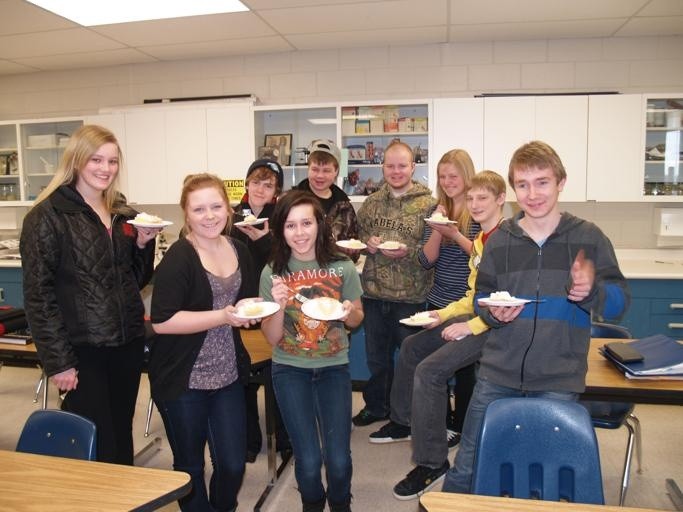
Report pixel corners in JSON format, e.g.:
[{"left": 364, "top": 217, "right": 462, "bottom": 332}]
[{"left": 598, "top": 334, "right": 683, "bottom": 377}]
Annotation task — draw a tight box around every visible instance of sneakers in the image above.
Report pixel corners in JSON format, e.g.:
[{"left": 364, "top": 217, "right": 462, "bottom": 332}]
[
  {"left": 446, "top": 429, "right": 461, "bottom": 452},
  {"left": 353, "top": 408, "right": 391, "bottom": 427},
  {"left": 369, "top": 422, "right": 411, "bottom": 444},
  {"left": 392, "top": 459, "right": 450, "bottom": 499}
]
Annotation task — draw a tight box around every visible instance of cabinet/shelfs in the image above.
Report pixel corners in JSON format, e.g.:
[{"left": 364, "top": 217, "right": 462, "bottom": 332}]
[
  {"left": 1, "top": 238, "right": 24, "bottom": 310},
  {"left": 1, "top": 93, "right": 683, "bottom": 206},
  {"left": 610, "top": 248, "right": 683, "bottom": 338}
]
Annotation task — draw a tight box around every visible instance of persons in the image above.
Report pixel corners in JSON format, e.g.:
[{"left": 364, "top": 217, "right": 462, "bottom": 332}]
[
  {"left": 291, "top": 138, "right": 361, "bottom": 264},
  {"left": 369, "top": 170, "right": 506, "bottom": 501},
  {"left": 352, "top": 141, "right": 436, "bottom": 427},
  {"left": 229, "top": 157, "right": 293, "bottom": 464},
  {"left": 441, "top": 140, "right": 631, "bottom": 494},
  {"left": 259, "top": 190, "right": 365, "bottom": 512},
  {"left": 147, "top": 174, "right": 262, "bottom": 511},
  {"left": 18, "top": 125, "right": 164, "bottom": 467},
  {"left": 417, "top": 147, "right": 481, "bottom": 452}
]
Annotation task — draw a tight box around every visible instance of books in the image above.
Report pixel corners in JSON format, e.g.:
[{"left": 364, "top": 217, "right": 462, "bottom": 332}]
[
  {"left": 0, "top": 327, "right": 33, "bottom": 345},
  {"left": 597, "top": 333, "right": 683, "bottom": 380}
]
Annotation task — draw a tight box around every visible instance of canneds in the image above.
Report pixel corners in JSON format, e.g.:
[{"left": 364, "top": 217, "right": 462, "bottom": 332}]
[
  {"left": 8, "top": 183, "right": 17, "bottom": 200},
  {"left": 0, "top": 183, "right": 11, "bottom": 200}
]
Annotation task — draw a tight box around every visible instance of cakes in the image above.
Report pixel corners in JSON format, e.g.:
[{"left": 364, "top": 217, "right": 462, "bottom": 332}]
[
  {"left": 409, "top": 311, "right": 429, "bottom": 322},
  {"left": 243, "top": 213, "right": 256, "bottom": 222},
  {"left": 383, "top": 241, "right": 398, "bottom": 247},
  {"left": 135, "top": 212, "right": 161, "bottom": 224},
  {"left": 429, "top": 213, "right": 450, "bottom": 223},
  {"left": 346, "top": 239, "right": 361, "bottom": 247},
  {"left": 244, "top": 300, "right": 263, "bottom": 316},
  {"left": 490, "top": 291, "right": 516, "bottom": 302},
  {"left": 316, "top": 296, "right": 337, "bottom": 314}
]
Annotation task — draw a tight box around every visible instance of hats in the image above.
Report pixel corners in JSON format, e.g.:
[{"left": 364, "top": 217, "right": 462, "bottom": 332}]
[
  {"left": 306, "top": 137, "right": 340, "bottom": 164},
  {"left": 244, "top": 159, "right": 283, "bottom": 195}
]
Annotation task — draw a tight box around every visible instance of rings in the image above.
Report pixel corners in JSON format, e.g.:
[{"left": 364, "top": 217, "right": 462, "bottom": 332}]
[{"left": 147, "top": 231, "right": 150, "bottom": 235}]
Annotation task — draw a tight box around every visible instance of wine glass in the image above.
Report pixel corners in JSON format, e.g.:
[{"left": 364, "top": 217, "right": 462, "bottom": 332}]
[{"left": 0, "top": 183, "right": 15, "bottom": 201}]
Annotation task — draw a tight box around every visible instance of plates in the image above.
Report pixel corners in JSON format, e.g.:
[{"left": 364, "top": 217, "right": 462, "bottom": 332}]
[
  {"left": 424, "top": 218, "right": 458, "bottom": 225},
  {"left": 232, "top": 301, "right": 280, "bottom": 319},
  {"left": 301, "top": 296, "right": 347, "bottom": 320},
  {"left": 126, "top": 220, "right": 172, "bottom": 228},
  {"left": 333, "top": 240, "right": 368, "bottom": 250},
  {"left": 399, "top": 317, "right": 436, "bottom": 326},
  {"left": 477, "top": 297, "right": 525, "bottom": 307},
  {"left": 232, "top": 218, "right": 268, "bottom": 227},
  {"left": 376, "top": 245, "right": 405, "bottom": 251}
]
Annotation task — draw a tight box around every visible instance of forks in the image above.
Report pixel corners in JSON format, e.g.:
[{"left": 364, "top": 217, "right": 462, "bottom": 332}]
[{"left": 268, "top": 274, "right": 309, "bottom": 304}]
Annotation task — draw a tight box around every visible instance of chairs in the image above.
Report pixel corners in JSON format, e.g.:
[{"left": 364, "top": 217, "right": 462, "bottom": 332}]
[
  {"left": 1, "top": 357, "right": 44, "bottom": 403},
  {"left": 15, "top": 408, "right": 98, "bottom": 461},
  {"left": 470, "top": 396, "right": 605, "bottom": 506},
  {"left": 578, "top": 322, "right": 644, "bottom": 509}
]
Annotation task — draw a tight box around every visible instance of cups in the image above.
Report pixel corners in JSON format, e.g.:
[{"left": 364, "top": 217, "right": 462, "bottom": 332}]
[
  {"left": 646, "top": 104, "right": 682, "bottom": 127},
  {"left": 45, "top": 164, "right": 54, "bottom": 174},
  {"left": 645, "top": 181, "right": 683, "bottom": 194}
]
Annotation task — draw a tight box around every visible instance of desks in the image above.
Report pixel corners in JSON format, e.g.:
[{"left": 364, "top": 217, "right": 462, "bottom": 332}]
[
  {"left": 578, "top": 339, "right": 683, "bottom": 405},
  {"left": 1, "top": 449, "right": 193, "bottom": 511},
  {"left": 1, "top": 328, "right": 294, "bottom": 511},
  {"left": 419, "top": 492, "right": 682, "bottom": 512}
]
